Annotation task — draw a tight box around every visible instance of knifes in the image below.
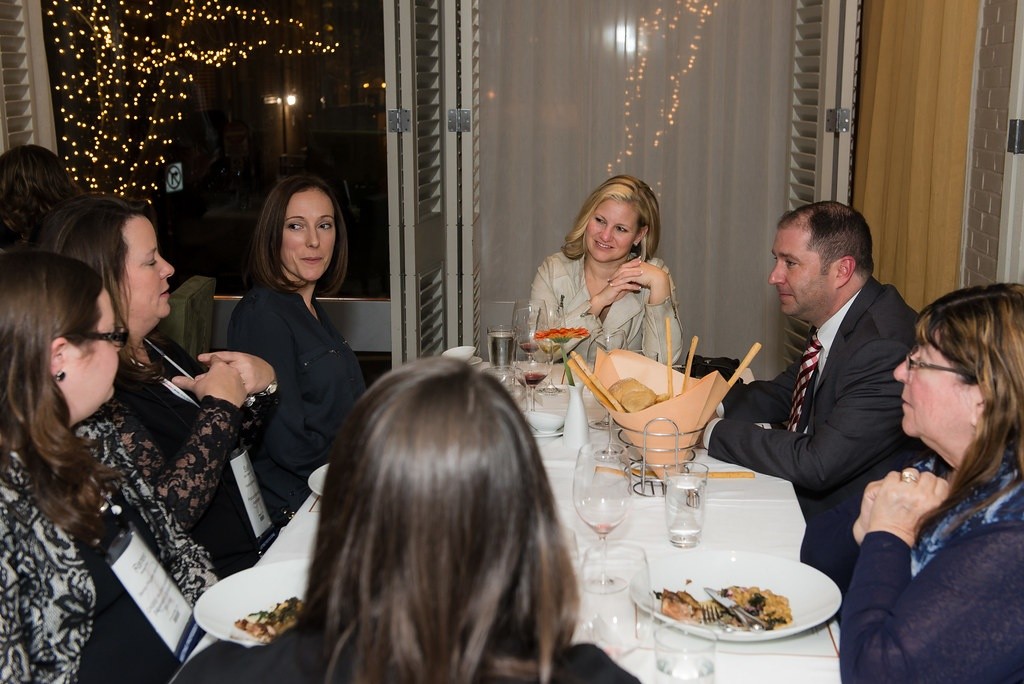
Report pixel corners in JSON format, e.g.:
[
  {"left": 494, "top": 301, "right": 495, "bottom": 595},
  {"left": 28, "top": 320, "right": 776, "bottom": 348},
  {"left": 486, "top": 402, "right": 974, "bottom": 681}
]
[{"left": 704, "top": 588, "right": 768, "bottom": 632}]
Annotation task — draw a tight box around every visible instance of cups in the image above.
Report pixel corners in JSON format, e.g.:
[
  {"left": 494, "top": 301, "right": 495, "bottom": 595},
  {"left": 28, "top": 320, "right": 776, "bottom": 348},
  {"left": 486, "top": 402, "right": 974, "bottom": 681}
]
[
  {"left": 575, "top": 542, "right": 654, "bottom": 662},
  {"left": 587, "top": 327, "right": 627, "bottom": 374},
  {"left": 487, "top": 324, "right": 518, "bottom": 370},
  {"left": 629, "top": 348, "right": 659, "bottom": 363},
  {"left": 665, "top": 462, "right": 709, "bottom": 548},
  {"left": 655, "top": 621, "right": 717, "bottom": 684}
]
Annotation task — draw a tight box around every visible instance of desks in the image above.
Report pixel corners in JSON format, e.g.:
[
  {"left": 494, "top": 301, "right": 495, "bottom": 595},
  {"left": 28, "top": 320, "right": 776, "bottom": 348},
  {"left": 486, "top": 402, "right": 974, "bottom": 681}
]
[{"left": 184, "top": 362, "right": 842, "bottom": 684}]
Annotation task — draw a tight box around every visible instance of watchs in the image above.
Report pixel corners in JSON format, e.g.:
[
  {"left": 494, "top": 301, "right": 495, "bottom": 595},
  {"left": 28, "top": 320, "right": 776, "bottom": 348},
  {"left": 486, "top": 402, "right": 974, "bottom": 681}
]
[{"left": 243, "top": 376, "right": 278, "bottom": 407}]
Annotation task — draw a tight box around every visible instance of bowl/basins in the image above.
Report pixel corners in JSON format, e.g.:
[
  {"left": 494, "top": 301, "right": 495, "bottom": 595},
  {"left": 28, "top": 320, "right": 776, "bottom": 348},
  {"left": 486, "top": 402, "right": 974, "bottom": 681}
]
[
  {"left": 441, "top": 345, "right": 476, "bottom": 364},
  {"left": 523, "top": 412, "right": 565, "bottom": 433}
]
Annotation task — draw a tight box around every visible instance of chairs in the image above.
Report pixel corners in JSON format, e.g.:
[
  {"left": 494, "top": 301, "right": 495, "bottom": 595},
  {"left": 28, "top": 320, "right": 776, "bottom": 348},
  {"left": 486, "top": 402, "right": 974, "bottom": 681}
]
[{"left": 169, "top": 276, "right": 217, "bottom": 360}]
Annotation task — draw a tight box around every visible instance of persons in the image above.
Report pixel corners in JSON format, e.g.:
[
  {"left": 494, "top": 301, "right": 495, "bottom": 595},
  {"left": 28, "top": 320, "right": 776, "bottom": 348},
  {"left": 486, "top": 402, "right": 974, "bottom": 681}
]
[
  {"left": 693, "top": 199, "right": 935, "bottom": 520},
  {"left": 0, "top": 144, "right": 368, "bottom": 684},
  {"left": 528, "top": 175, "right": 683, "bottom": 369},
  {"left": 800, "top": 283, "right": 1024, "bottom": 684},
  {"left": 168, "top": 355, "right": 642, "bottom": 684}
]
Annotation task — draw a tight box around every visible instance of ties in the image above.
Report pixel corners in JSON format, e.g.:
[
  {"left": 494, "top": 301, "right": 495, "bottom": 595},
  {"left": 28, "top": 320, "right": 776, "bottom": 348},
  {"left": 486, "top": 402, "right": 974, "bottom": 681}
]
[{"left": 784, "top": 328, "right": 822, "bottom": 431}]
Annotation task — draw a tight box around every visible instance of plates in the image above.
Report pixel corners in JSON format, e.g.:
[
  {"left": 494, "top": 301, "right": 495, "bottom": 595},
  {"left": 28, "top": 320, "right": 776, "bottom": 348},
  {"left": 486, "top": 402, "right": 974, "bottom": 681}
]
[
  {"left": 193, "top": 558, "right": 310, "bottom": 650},
  {"left": 633, "top": 549, "right": 842, "bottom": 641},
  {"left": 468, "top": 356, "right": 483, "bottom": 365},
  {"left": 530, "top": 427, "right": 564, "bottom": 438},
  {"left": 308, "top": 463, "right": 329, "bottom": 495}
]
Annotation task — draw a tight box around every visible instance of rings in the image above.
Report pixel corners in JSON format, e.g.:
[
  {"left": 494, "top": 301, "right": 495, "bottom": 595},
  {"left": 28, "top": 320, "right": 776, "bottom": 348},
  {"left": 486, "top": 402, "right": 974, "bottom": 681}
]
[{"left": 902, "top": 472, "right": 918, "bottom": 483}]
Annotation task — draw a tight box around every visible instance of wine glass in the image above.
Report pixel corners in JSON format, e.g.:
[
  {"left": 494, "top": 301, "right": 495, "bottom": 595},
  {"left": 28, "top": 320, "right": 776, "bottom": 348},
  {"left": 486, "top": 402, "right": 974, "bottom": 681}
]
[
  {"left": 573, "top": 444, "right": 632, "bottom": 594},
  {"left": 511, "top": 299, "right": 558, "bottom": 425}
]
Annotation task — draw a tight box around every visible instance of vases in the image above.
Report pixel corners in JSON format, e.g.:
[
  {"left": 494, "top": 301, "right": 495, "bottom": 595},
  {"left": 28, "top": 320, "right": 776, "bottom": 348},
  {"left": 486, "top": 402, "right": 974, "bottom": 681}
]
[{"left": 564, "top": 383, "right": 590, "bottom": 451}]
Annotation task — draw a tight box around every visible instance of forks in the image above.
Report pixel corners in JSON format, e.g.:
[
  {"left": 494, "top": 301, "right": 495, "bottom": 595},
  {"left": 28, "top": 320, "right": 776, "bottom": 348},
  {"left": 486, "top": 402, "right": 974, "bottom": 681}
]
[{"left": 703, "top": 604, "right": 734, "bottom": 633}]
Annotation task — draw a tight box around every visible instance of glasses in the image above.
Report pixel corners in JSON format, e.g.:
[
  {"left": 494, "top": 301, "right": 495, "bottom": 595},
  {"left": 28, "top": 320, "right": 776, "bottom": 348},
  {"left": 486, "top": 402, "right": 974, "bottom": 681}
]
[
  {"left": 906, "top": 344, "right": 983, "bottom": 374},
  {"left": 63, "top": 325, "right": 130, "bottom": 346}
]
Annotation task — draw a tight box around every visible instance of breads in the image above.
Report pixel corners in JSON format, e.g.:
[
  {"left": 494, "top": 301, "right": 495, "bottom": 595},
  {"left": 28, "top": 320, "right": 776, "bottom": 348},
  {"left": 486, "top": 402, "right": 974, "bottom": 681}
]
[{"left": 608, "top": 378, "right": 662, "bottom": 415}]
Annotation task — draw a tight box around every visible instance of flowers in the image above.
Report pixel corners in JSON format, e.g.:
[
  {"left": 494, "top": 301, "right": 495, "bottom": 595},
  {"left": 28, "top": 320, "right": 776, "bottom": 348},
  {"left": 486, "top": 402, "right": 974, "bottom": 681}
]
[{"left": 534, "top": 327, "right": 591, "bottom": 387}]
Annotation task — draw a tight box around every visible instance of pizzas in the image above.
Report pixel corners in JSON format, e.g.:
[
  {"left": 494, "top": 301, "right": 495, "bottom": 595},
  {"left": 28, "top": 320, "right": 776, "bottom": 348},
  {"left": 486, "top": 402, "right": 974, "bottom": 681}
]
[{"left": 234, "top": 597, "right": 305, "bottom": 643}]
